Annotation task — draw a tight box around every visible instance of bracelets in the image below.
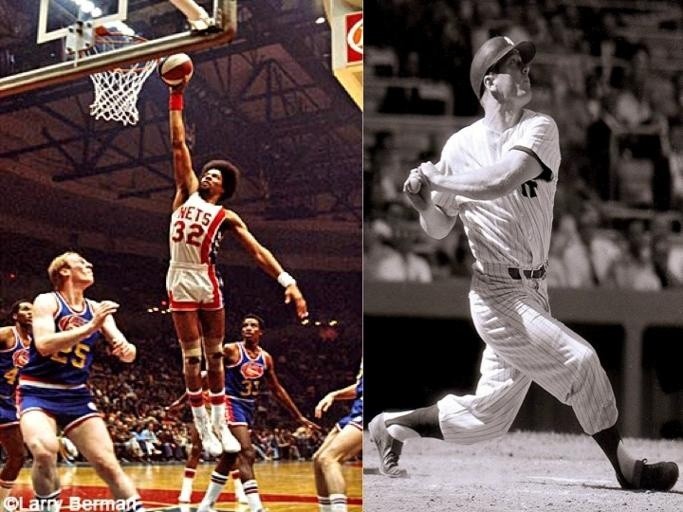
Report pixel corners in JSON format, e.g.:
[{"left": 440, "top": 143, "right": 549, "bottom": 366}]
[{"left": 276, "top": 271, "right": 298, "bottom": 289}]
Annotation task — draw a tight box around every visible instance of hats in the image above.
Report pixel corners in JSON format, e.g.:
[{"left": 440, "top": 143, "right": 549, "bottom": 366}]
[{"left": 470, "top": 35, "right": 536, "bottom": 101}]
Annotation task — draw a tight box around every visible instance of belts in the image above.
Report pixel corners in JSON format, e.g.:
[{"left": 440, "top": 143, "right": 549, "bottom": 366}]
[{"left": 509, "top": 263, "right": 545, "bottom": 281}]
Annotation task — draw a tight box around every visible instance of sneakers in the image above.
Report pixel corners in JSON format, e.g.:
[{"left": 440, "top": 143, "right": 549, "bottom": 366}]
[
  {"left": 193, "top": 418, "right": 241, "bottom": 457},
  {"left": 616, "top": 459, "right": 679, "bottom": 491},
  {"left": 197, "top": 504, "right": 218, "bottom": 512},
  {"left": 235, "top": 490, "right": 248, "bottom": 504},
  {"left": 367, "top": 410, "right": 407, "bottom": 478},
  {"left": 178, "top": 486, "right": 192, "bottom": 502},
  {"left": 120, "top": 453, "right": 314, "bottom": 465}
]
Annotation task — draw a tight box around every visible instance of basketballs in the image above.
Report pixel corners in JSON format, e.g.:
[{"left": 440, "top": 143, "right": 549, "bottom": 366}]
[{"left": 156, "top": 52, "right": 194, "bottom": 87}]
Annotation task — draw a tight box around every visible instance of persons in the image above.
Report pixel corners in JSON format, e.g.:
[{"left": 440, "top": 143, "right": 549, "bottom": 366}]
[
  {"left": 368, "top": 34, "right": 678, "bottom": 492},
  {"left": 16, "top": 250, "right": 148, "bottom": 512},
  {"left": 361, "top": 1, "right": 682, "bottom": 292},
  {"left": 310, "top": 354, "right": 363, "bottom": 512},
  {"left": 164, "top": 311, "right": 329, "bottom": 512},
  {"left": 177, "top": 369, "right": 249, "bottom": 506},
  {"left": 162, "top": 75, "right": 309, "bottom": 458},
  {"left": 0, "top": 298, "right": 35, "bottom": 509}
]
